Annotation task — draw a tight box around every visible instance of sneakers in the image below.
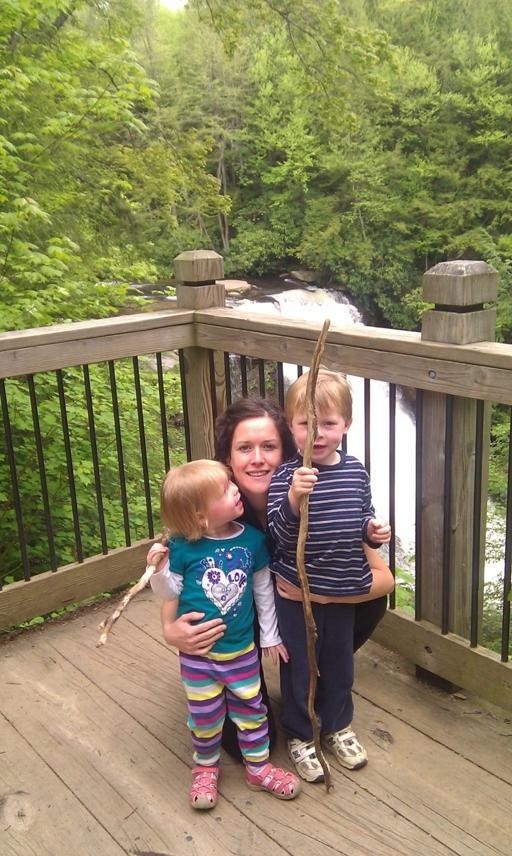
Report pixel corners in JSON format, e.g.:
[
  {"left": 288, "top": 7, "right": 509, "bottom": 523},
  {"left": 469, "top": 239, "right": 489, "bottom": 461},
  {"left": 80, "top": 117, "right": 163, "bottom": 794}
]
[
  {"left": 287, "top": 738, "right": 324, "bottom": 782},
  {"left": 190, "top": 766, "right": 219, "bottom": 810},
  {"left": 246, "top": 763, "right": 301, "bottom": 800},
  {"left": 321, "top": 724, "right": 367, "bottom": 770}
]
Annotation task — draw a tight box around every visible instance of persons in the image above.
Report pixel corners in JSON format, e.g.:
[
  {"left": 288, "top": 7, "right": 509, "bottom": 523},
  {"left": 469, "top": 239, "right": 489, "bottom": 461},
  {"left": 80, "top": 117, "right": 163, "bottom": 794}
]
[
  {"left": 159, "top": 395, "right": 394, "bottom": 770},
  {"left": 264, "top": 369, "right": 394, "bottom": 786},
  {"left": 144, "top": 457, "right": 302, "bottom": 811}
]
